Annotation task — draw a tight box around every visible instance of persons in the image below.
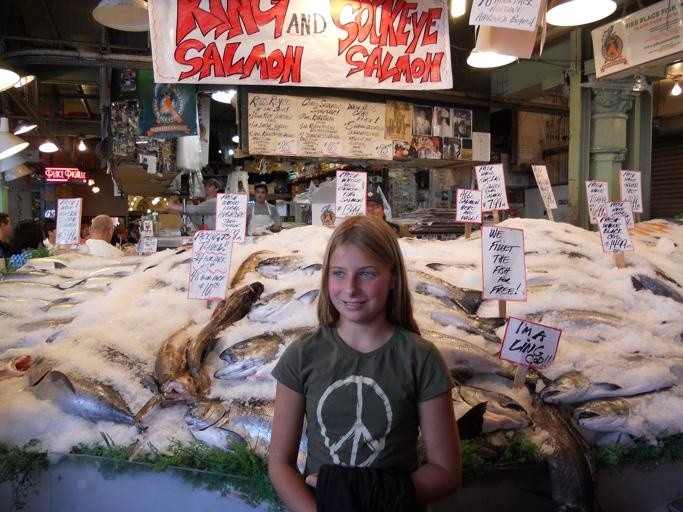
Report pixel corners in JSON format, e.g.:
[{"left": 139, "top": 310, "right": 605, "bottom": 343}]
[
  {"left": 0, "top": 198, "right": 56, "bottom": 271},
  {"left": 264, "top": 215, "right": 461, "bottom": 512},
  {"left": 84, "top": 214, "right": 125, "bottom": 259},
  {"left": 238, "top": 181, "right": 247, "bottom": 195},
  {"left": 246, "top": 184, "right": 279, "bottom": 235},
  {"left": 169, "top": 179, "right": 221, "bottom": 230},
  {"left": 365, "top": 190, "right": 401, "bottom": 234},
  {"left": 130, "top": 226, "right": 139, "bottom": 242}
]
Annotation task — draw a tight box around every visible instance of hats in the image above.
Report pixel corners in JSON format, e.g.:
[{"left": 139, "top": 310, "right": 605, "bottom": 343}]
[
  {"left": 368, "top": 191, "right": 382, "bottom": 203},
  {"left": 208, "top": 179, "right": 220, "bottom": 189},
  {"left": 254, "top": 181, "right": 267, "bottom": 188}
]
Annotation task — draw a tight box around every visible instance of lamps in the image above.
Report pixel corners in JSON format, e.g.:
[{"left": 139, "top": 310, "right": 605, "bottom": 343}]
[
  {"left": 78, "top": 139, "right": 87, "bottom": 152},
  {"left": 38, "top": 139, "right": 59, "bottom": 153},
  {"left": 14, "top": 119, "right": 38, "bottom": 136},
  {"left": 467, "top": 29, "right": 518, "bottom": 69},
  {"left": 14, "top": 72, "right": 36, "bottom": 88},
  {"left": 4, "top": 164, "right": 33, "bottom": 182},
  {"left": 0, "top": 64, "right": 19, "bottom": 92},
  {"left": 92, "top": 0, "right": 150, "bottom": 32},
  {"left": 0, "top": 154, "right": 27, "bottom": 174},
  {"left": 0, "top": 117, "right": 30, "bottom": 160},
  {"left": 544, "top": 0, "right": 618, "bottom": 28}
]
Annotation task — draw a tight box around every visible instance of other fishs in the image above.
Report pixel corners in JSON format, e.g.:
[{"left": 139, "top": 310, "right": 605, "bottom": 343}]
[
  {"left": 184, "top": 400, "right": 489, "bottom": 475},
  {"left": 247, "top": 288, "right": 509, "bottom": 343},
  {"left": 0, "top": 216, "right": 683, "bottom": 466},
  {"left": 29, "top": 356, "right": 149, "bottom": 434},
  {"left": 529, "top": 391, "right": 594, "bottom": 512},
  {"left": 189, "top": 282, "right": 264, "bottom": 393},
  {"left": 214, "top": 325, "right": 504, "bottom": 381},
  {"left": 539, "top": 355, "right": 683, "bottom": 406},
  {"left": 254, "top": 254, "right": 482, "bottom": 318},
  {"left": 571, "top": 386, "right": 683, "bottom": 440}
]
